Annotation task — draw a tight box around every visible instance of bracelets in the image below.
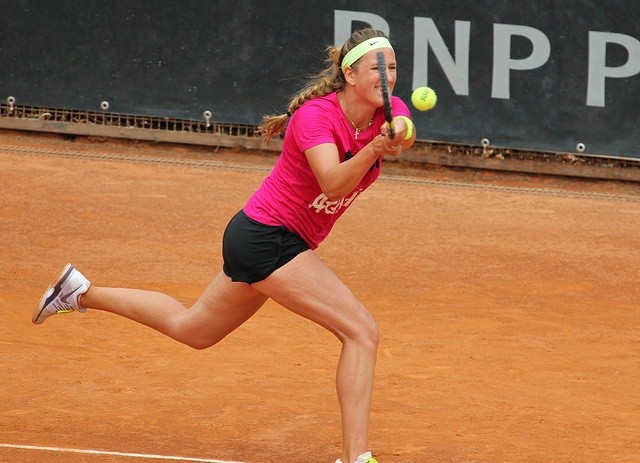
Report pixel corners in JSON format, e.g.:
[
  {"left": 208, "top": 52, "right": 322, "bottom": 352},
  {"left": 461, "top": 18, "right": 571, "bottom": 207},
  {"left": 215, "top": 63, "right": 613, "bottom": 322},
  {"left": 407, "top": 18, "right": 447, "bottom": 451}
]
[{"left": 393, "top": 115, "right": 414, "bottom": 142}]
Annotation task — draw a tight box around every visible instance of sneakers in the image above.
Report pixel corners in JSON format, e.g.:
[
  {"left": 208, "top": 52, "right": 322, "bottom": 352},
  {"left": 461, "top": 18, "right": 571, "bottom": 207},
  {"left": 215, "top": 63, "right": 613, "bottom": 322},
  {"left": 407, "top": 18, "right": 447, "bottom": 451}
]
[
  {"left": 336, "top": 452, "right": 377, "bottom": 463},
  {"left": 32, "top": 263, "right": 91, "bottom": 325}
]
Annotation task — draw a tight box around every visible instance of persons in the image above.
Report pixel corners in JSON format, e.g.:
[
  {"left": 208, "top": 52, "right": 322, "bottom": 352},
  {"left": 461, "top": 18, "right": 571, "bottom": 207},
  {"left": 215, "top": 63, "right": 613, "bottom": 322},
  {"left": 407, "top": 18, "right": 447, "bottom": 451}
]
[{"left": 32, "top": 29, "right": 417, "bottom": 463}]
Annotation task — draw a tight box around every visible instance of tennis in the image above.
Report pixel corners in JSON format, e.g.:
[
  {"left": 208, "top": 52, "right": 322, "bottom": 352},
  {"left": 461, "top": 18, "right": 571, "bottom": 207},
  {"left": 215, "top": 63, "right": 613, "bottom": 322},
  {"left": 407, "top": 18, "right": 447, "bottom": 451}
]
[{"left": 412, "top": 87, "right": 436, "bottom": 112}]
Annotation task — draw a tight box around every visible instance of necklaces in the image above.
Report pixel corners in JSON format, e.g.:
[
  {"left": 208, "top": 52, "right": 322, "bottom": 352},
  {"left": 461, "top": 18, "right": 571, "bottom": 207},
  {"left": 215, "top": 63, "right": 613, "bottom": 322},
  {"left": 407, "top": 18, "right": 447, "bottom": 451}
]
[{"left": 339, "top": 90, "right": 374, "bottom": 140}]
